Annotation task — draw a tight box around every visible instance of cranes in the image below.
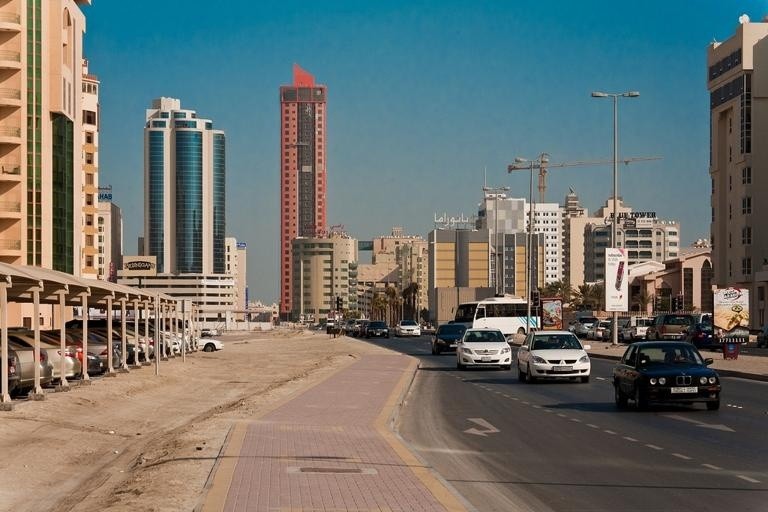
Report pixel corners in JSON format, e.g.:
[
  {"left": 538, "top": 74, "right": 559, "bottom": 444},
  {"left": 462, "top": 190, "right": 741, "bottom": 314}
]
[{"left": 507, "top": 152, "right": 664, "bottom": 203}]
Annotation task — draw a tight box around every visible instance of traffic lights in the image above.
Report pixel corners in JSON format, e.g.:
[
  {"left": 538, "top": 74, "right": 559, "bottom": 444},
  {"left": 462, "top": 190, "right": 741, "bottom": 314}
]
[{"left": 337, "top": 297, "right": 343, "bottom": 310}]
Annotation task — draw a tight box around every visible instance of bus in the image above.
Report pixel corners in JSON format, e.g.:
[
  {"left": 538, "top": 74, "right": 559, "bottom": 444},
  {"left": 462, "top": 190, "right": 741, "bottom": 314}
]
[{"left": 454, "top": 297, "right": 540, "bottom": 334}]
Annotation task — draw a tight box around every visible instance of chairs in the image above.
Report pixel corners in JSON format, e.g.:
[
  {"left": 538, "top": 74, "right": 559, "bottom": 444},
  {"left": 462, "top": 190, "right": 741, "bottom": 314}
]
[{"left": 630, "top": 352, "right": 652, "bottom": 367}]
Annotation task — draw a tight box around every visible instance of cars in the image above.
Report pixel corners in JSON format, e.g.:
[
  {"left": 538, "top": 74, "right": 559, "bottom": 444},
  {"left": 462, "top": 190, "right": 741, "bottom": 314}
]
[
  {"left": 567, "top": 313, "right": 712, "bottom": 347},
  {"left": 431, "top": 323, "right": 721, "bottom": 411},
  {"left": 338, "top": 319, "right": 421, "bottom": 339},
  {"left": 757, "top": 324, "right": 768, "bottom": 347},
  {"left": 0, "top": 320, "right": 224, "bottom": 397}
]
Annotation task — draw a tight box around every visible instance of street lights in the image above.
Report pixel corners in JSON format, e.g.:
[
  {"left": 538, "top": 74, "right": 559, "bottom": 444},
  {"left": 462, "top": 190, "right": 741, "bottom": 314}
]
[
  {"left": 398, "top": 296, "right": 403, "bottom": 320},
  {"left": 591, "top": 91, "right": 641, "bottom": 347},
  {"left": 514, "top": 157, "right": 549, "bottom": 334},
  {"left": 483, "top": 186, "right": 511, "bottom": 296}
]
